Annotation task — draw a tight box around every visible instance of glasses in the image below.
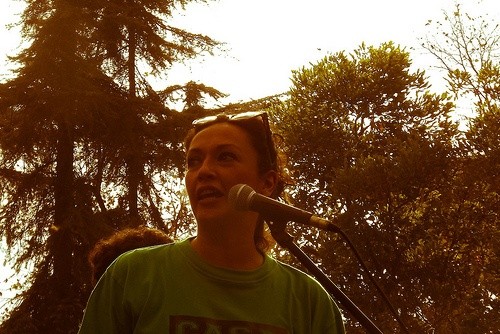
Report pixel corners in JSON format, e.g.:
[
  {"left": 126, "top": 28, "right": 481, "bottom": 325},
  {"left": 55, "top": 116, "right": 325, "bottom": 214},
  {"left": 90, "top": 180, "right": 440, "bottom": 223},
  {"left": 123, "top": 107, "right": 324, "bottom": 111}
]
[{"left": 192, "top": 111, "right": 277, "bottom": 170}]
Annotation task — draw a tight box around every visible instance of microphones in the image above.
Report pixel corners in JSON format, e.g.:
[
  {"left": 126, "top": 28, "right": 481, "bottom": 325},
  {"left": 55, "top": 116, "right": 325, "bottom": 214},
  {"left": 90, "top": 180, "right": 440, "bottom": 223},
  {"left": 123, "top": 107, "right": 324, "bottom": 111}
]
[{"left": 228, "top": 184, "right": 340, "bottom": 233}]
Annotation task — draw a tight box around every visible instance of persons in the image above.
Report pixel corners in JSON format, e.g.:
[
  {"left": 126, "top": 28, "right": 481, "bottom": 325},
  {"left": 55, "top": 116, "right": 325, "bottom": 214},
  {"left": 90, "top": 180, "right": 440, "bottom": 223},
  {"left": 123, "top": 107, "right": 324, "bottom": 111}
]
[{"left": 78, "top": 111, "right": 347, "bottom": 334}]
